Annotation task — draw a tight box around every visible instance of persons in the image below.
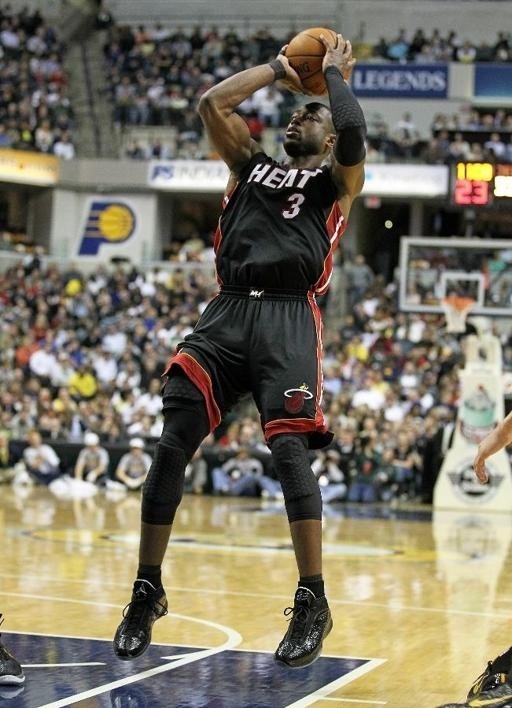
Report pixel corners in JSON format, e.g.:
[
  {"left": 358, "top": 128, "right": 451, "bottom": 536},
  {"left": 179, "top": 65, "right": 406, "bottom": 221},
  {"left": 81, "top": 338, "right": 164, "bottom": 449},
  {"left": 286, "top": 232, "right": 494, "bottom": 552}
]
[
  {"left": 465, "top": 646, "right": 512, "bottom": 708},
  {"left": 0, "top": 201, "right": 512, "bottom": 504},
  {"left": 1, "top": 0, "right": 512, "bottom": 163},
  {"left": 0, "top": 642, "right": 26, "bottom": 686},
  {"left": 113, "top": 33, "right": 367, "bottom": 669}
]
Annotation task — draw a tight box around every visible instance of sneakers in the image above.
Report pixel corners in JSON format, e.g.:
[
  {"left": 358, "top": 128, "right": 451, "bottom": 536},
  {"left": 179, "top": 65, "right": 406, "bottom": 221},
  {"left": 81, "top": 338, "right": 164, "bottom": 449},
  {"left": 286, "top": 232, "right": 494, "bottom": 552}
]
[
  {"left": 1, "top": 612, "right": 26, "bottom": 685},
  {"left": 274, "top": 587, "right": 333, "bottom": 670},
  {"left": 114, "top": 579, "right": 168, "bottom": 661},
  {"left": 466, "top": 655, "right": 512, "bottom": 707}
]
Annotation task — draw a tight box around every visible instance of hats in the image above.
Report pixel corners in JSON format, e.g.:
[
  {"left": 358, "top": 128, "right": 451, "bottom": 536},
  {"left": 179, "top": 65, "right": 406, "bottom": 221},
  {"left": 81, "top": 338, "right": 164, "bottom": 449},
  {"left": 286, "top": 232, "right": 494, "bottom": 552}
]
[
  {"left": 84, "top": 432, "right": 99, "bottom": 447},
  {"left": 129, "top": 437, "right": 146, "bottom": 449}
]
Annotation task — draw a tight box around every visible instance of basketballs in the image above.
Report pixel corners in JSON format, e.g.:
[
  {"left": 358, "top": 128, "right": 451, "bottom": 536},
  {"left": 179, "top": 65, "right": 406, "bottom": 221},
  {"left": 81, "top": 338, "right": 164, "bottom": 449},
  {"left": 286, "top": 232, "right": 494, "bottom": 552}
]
[{"left": 286, "top": 27, "right": 351, "bottom": 96}]
